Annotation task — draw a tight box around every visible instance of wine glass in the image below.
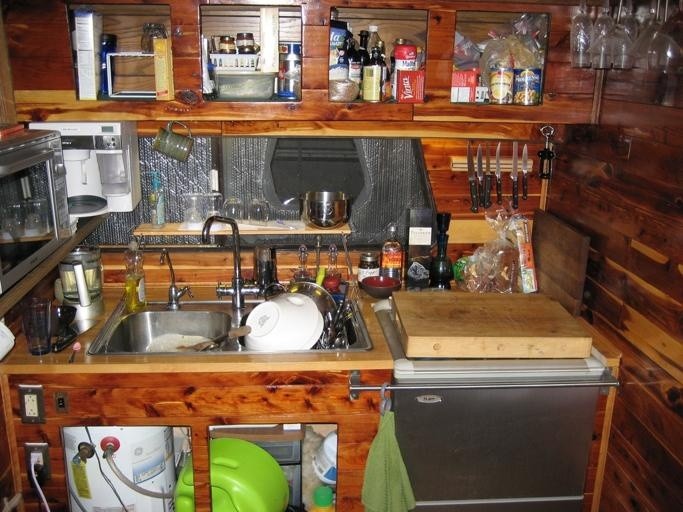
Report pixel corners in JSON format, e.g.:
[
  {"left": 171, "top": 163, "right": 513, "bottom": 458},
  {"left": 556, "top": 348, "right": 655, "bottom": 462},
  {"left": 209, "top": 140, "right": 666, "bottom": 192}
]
[{"left": 568, "top": 0, "right": 683, "bottom": 75}]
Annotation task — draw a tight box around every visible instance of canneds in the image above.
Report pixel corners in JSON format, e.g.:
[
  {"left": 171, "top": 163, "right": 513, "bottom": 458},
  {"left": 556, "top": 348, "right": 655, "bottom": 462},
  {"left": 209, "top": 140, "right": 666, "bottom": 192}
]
[
  {"left": 140, "top": 22, "right": 165, "bottom": 54},
  {"left": 278, "top": 41, "right": 302, "bottom": 100},
  {"left": 391, "top": 39, "right": 417, "bottom": 104},
  {"left": 357, "top": 252, "right": 380, "bottom": 287},
  {"left": 514, "top": 68, "right": 542, "bottom": 105},
  {"left": 218, "top": 37, "right": 236, "bottom": 54},
  {"left": 236, "top": 33, "right": 254, "bottom": 53},
  {"left": 488, "top": 67, "right": 513, "bottom": 103}
]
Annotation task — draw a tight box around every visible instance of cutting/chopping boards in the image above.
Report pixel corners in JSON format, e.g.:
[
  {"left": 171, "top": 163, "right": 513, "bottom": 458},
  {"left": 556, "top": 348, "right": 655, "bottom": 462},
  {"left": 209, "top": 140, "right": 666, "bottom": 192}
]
[
  {"left": 529, "top": 206, "right": 592, "bottom": 317},
  {"left": 387, "top": 290, "right": 594, "bottom": 360}
]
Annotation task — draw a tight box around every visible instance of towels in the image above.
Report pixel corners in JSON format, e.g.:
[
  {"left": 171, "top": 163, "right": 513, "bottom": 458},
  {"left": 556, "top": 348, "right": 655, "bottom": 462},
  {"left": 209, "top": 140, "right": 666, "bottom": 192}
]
[{"left": 360, "top": 411, "right": 416, "bottom": 512}]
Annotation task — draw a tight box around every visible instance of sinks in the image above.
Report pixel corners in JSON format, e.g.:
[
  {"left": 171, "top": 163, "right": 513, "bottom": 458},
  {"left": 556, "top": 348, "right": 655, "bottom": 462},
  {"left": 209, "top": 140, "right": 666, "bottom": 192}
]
[
  {"left": 235, "top": 302, "right": 372, "bottom": 352},
  {"left": 87, "top": 301, "right": 234, "bottom": 355}
]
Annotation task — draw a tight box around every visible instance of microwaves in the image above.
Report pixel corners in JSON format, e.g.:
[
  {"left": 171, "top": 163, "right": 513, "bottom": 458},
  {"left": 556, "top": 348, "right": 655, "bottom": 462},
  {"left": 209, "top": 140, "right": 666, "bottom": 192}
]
[{"left": 0, "top": 126, "right": 72, "bottom": 296}]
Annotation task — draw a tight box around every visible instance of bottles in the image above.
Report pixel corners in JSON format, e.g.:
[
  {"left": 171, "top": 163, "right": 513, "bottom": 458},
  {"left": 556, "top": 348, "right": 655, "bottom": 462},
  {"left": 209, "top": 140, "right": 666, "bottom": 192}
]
[
  {"left": 307, "top": 485, "right": 335, "bottom": 511},
  {"left": 138, "top": 19, "right": 167, "bottom": 55},
  {"left": 347, "top": 25, "right": 406, "bottom": 102},
  {"left": 432, "top": 211, "right": 454, "bottom": 288},
  {"left": 292, "top": 243, "right": 312, "bottom": 286},
  {"left": 380, "top": 221, "right": 403, "bottom": 287},
  {"left": 324, "top": 242, "right": 344, "bottom": 293}
]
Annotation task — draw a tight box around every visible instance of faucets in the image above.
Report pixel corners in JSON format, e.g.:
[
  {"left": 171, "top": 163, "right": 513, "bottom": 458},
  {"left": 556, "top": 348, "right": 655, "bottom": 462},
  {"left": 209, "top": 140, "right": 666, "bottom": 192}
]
[
  {"left": 159, "top": 249, "right": 195, "bottom": 310},
  {"left": 201, "top": 215, "right": 264, "bottom": 308}
]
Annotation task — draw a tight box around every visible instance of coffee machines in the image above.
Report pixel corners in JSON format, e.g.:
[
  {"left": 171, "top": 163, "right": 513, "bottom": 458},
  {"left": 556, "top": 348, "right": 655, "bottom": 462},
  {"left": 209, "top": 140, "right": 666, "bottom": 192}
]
[
  {"left": 28, "top": 122, "right": 143, "bottom": 217},
  {"left": 58, "top": 246, "right": 105, "bottom": 335}
]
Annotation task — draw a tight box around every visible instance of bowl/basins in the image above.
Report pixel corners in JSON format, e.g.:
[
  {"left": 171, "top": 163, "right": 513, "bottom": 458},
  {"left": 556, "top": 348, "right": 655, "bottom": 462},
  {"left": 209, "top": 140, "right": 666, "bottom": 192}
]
[
  {"left": 311, "top": 428, "right": 336, "bottom": 482},
  {"left": 144, "top": 333, "right": 221, "bottom": 352},
  {"left": 243, "top": 283, "right": 334, "bottom": 350},
  {"left": 297, "top": 190, "right": 353, "bottom": 230},
  {"left": 174, "top": 436, "right": 289, "bottom": 512},
  {"left": 53, "top": 306, "right": 76, "bottom": 324}
]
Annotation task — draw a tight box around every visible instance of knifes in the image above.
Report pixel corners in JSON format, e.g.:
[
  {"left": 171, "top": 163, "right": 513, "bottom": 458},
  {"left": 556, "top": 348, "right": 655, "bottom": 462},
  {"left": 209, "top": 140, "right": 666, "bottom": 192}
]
[{"left": 465, "top": 139, "right": 528, "bottom": 212}]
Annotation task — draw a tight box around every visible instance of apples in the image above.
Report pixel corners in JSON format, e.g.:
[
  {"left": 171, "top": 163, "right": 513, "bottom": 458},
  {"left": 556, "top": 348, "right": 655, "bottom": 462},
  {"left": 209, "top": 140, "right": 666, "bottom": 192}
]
[{"left": 324, "top": 276, "right": 340, "bottom": 291}]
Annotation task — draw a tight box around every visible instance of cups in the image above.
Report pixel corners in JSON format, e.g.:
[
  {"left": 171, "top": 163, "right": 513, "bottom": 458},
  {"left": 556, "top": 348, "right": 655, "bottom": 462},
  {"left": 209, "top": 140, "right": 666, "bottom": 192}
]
[
  {"left": 21, "top": 297, "right": 52, "bottom": 357},
  {"left": 181, "top": 191, "right": 270, "bottom": 228},
  {"left": 152, "top": 120, "right": 195, "bottom": 162},
  {"left": 98, "top": 31, "right": 115, "bottom": 93}
]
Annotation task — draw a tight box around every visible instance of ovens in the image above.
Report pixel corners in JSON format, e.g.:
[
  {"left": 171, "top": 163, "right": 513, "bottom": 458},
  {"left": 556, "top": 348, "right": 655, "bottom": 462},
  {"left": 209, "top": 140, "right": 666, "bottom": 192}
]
[{"left": 393, "top": 365, "right": 623, "bottom": 511}]
